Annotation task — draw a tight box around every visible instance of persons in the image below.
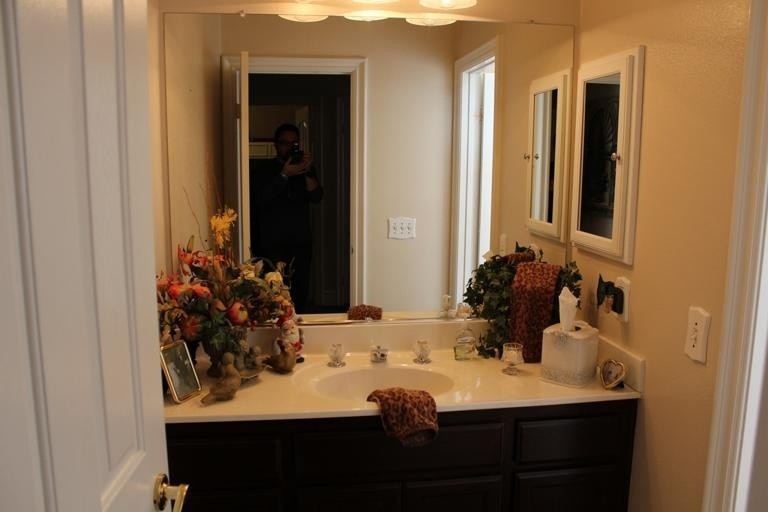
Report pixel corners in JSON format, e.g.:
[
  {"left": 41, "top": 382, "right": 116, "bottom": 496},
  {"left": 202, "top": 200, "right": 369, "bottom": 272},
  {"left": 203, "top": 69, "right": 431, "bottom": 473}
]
[
  {"left": 596, "top": 287, "right": 617, "bottom": 316},
  {"left": 277, "top": 314, "right": 306, "bottom": 364},
  {"left": 200, "top": 352, "right": 241, "bottom": 405},
  {"left": 250, "top": 124, "right": 324, "bottom": 314}
]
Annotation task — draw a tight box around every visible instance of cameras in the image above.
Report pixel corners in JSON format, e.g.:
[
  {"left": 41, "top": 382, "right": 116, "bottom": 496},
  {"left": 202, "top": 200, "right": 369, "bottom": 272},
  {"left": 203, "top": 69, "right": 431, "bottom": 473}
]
[{"left": 290, "top": 142, "right": 304, "bottom": 164}]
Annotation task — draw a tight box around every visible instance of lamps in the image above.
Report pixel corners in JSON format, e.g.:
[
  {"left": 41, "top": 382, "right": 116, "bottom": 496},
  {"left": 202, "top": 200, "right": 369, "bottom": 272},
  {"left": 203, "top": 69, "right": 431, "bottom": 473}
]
[
  {"left": 419, "top": 0, "right": 477, "bottom": 11},
  {"left": 279, "top": 13, "right": 456, "bottom": 27}
]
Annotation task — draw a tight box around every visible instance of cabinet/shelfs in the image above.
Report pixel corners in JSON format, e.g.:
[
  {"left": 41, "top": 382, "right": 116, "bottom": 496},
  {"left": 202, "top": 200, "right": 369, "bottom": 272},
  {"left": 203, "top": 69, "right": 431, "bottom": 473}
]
[
  {"left": 571, "top": 43, "right": 646, "bottom": 266},
  {"left": 164, "top": 397, "right": 637, "bottom": 512},
  {"left": 525, "top": 67, "right": 571, "bottom": 245}
]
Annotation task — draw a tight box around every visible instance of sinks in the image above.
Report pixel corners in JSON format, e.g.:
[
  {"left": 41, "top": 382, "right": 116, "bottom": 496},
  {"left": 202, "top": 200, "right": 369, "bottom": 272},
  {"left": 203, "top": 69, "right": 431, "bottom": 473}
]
[{"left": 293, "top": 353, "right": 479, "bottom": 406}]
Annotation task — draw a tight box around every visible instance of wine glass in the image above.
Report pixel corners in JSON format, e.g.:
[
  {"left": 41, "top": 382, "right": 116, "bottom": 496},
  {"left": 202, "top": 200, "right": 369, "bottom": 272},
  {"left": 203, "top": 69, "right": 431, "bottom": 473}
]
[
  {"left": 326, "top": 344, "right": 348, "bottom": 368},
  {"left": 413, "top": 340, "right": 433, "bottom": 366},
  {"left": 501, "top": 342, "right": 525, "bottom": 376}
]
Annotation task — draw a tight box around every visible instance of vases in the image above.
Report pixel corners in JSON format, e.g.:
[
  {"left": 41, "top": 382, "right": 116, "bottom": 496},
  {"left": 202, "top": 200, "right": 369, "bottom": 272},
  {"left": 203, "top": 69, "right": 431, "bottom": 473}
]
[{"left": 201, "top": 327, "right": 247, "bottom": 377}]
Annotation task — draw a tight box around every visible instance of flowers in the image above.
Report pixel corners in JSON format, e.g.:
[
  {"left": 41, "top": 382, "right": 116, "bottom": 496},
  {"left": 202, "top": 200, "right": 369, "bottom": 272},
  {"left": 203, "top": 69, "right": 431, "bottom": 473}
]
[
  {"left": 177, "top": 203, "right": 296, "bottom": 298},
  {"left": 155, "top": 182, "right": 289, "bottom": 349}
]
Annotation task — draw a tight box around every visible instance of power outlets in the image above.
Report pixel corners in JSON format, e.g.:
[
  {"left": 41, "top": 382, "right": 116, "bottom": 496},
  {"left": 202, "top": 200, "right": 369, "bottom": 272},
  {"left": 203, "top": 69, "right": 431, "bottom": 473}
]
[
  {"left": 684, "top": 306, "right": 712, "bottom": 364},
  {"left": 610, "top": 275, "right": 631, "bottom": 324},
  {"left": 500, "top": 233, "right": 507, "bottom": 257},
  {"left": 389, "top": 216, "right": 415, "bottom": 239}
]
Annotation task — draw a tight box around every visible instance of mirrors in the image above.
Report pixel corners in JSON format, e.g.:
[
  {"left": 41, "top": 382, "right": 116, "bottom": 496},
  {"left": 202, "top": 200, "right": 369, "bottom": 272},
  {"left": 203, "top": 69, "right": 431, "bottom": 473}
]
[{"left": 162, "top": 11, "right": 574, "bottom": 325}]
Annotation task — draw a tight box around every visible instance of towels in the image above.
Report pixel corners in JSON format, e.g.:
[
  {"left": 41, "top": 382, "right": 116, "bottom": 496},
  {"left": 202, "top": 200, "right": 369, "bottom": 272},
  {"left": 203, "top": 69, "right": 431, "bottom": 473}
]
[{"left": 366, "top": 387, "right": 440, "bottom": 448}]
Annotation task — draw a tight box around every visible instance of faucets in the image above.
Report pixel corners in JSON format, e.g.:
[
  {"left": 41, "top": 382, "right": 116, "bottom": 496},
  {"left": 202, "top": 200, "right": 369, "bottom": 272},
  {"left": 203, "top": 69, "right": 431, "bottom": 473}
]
[{"left": 370, "top": 345, "right": 389, "bottom": 363}]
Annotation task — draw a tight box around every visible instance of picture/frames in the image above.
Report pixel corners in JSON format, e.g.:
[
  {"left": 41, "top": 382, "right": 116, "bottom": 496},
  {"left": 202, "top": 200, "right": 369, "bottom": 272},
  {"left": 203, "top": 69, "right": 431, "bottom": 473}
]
[{"left": 159, "top": 339, "right": 201, "bottom": 403}]
